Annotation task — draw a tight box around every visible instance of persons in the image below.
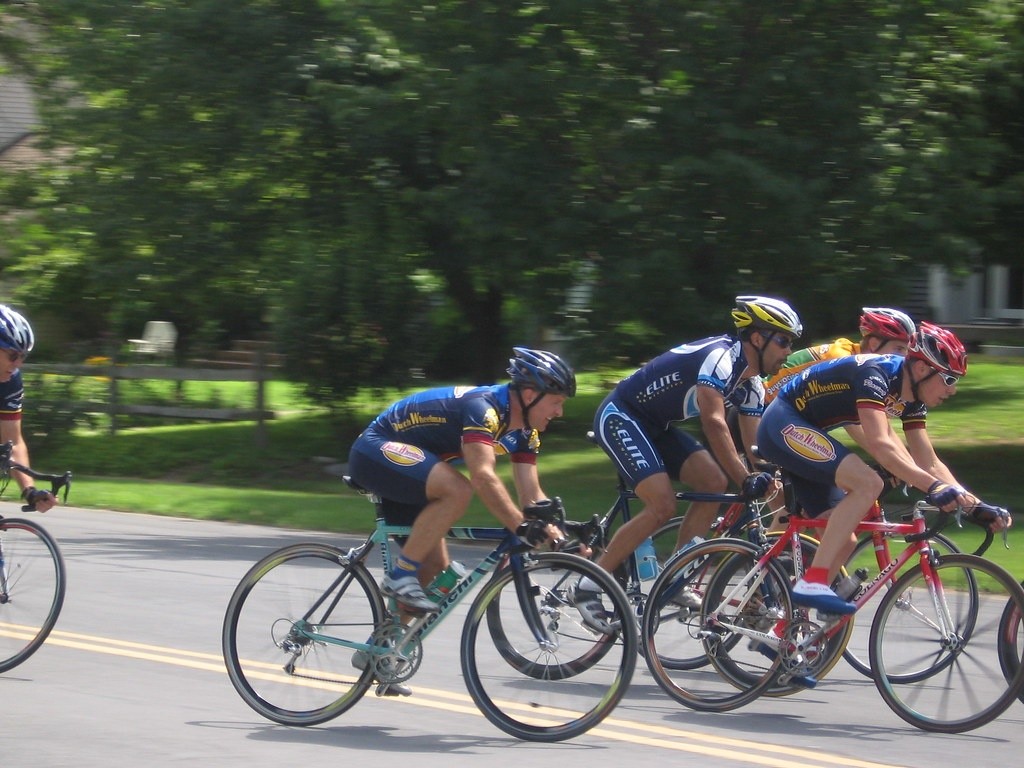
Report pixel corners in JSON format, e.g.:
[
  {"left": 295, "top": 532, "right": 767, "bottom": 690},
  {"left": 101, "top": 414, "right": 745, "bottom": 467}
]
[
  {"left": 567, "top": 295, "right": 802, "bottom": 635},
  {"left": 757, "top": 307, "right": 917, "bottom": 621},
  {"left": 748, "top": 321, "right": 1012, "bottom": 688},
  {"left": 0, "top": 304, "right": 60, "bottom": 514},
  {"left": 348, "top": 348, "right": 592, "bottom": 697}
]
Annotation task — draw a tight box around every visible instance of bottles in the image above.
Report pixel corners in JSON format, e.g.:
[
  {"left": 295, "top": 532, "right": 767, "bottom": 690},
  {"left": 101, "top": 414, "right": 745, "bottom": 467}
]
[
  {"left": 423, "top": 562, "right": 467, "bottom": 604},
  {"left": 664, "top": 536, "right": 707, "bottom": 567},
  {"left": 833, "top": 568, "right": 868, "bottom": 600},
  {"left": 635, "top": 535, "right": 658, "bottom": 580}
]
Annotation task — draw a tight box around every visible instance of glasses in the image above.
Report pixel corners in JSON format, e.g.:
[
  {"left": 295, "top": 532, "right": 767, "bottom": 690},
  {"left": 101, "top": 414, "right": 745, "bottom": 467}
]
[
  {"left": 1, "top": 348, "right": 26, "bottom": 363},
  {"left": 929, "top": 365, "right": 961, "bottom": 388},
  {"left": 759, "top": 332, "right": 794, "bottom": 349}
]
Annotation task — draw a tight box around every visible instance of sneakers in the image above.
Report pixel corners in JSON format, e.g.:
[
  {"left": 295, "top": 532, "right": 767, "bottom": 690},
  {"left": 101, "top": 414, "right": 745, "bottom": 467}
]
[
  {"left": 353, "top": 651, "right": 413, "bottom": 696},
  {"left": 759, "top": 643, "right": 818, "bottom": 690},
  {"left": 791, "top": 581, "right": 857, "bottom": 614},
  {"left": 381, "top": 571, "right": 438, "bottom": 612},
  {"left": 670, "top": 590, "right": 704, "bottom": 612},
  {"left": 567, "top": 584, "right": 614, "bottom": 638}
]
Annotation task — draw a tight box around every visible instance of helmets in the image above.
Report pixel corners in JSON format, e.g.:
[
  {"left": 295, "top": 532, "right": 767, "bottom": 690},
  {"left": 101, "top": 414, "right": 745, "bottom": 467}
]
[
  {"left": 905, "top": 321, "right": 966, "bottom": 377},
  {"left": 732, "top": 295, "right": 802, "bottom": 341},
  {"left": 506, "top": 347, "right": 576, "bottom": 401},
  {"left": 0, "top": 305, "right": 34, "bottom": 355},
  {"left": 859, "top": 307, "right": 917, "bottom": 343}
]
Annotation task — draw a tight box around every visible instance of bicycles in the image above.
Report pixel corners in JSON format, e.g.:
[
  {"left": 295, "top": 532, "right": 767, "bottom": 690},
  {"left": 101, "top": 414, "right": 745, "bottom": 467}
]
[
  {"left": 485, "top": 428, "right": 795, "bottom": 713},
  {"left": 699, "top": 458, "right": 1024, "bottom": 734},
  {"left": 222, "top": 474, "right": 638, "bottom": 743},
  {"left": 0, "top": 439, "right": 72, "bottom": 672},
  {"left": 611, "top": 444, "right": 1010, "bottom": 684},
  {"left": 997, "top": 580, "right": 1024, "bottom": 706}
]
